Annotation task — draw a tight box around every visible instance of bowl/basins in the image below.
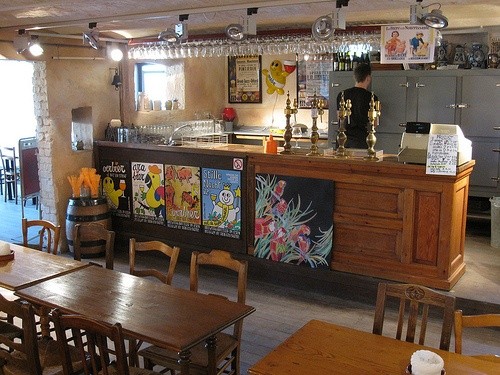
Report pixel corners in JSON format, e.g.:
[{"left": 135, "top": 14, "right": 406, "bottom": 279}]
[{"left": 406, "top": 363, "right": 446, "bottom": 375}]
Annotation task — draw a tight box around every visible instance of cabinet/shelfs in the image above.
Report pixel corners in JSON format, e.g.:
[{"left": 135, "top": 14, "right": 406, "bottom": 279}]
[{"left": 329, "top": 70, "right": 500, "bottom": 198}]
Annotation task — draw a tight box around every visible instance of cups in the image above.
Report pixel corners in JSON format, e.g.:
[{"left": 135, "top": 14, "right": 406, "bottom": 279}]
[
  {"left": 224, "top": 107, "right": 235, "bottom": 122},
  {"left": 118, "top": 118, "right": 224, "bottom": 145}
]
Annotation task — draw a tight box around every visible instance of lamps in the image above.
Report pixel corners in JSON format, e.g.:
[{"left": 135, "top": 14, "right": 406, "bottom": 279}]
[
  {"left": 410, "top": 0, "right": 448, "bottom": 29},
  {"left": 312, "top": 0, "right": 348, "bottom": 40},
  {"left": 158, "top": 14, "right": 189, "bottom": 43},
  {"left": 13, "top": 28, "right": 44, "bottom": 57},
  {"left": 83, "top": 22, "right": 99, "bottom": 50},
  {"left": 225, "top": 8, "right": 258, "bottom": 41}
]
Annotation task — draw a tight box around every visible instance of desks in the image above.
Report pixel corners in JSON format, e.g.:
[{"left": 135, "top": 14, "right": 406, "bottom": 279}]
[
  {"left": 248, "top": 319, "right": 500, "bottom": 375},
  {"left": 0, "top": 240, "right": 89, "bottom": 291},
  {"left": 13, "top": 265, "right": 256, "bottom": 375}
]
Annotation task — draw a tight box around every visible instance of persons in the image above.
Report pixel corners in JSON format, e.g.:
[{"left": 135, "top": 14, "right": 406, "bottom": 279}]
[{"left": 337, "top": 63, "right": 379, "bottom": 148}]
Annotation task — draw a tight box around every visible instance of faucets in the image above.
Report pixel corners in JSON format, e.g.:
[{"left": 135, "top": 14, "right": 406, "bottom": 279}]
[{"left": 164, "top": 124, "right": 193, "bottom": 146}]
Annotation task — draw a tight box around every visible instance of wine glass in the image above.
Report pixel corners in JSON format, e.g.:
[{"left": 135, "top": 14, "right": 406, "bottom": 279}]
[{"left": 128, "top": 30, "right": 380, "bottom": 60}]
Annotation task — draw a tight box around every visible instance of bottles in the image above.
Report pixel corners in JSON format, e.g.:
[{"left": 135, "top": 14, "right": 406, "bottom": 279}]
[
  {"left": 266, "top": 134, "right": 277, "bottom": 155},
  {"left": 137, "top": 92, "right": 178, "bottom": 110},
  {"left": 334, "top": 52, "right": 370, "bottom": 71},
  {"left": 436, "top": 40, "right": 449, "bottom": 67},
  {"left": 467, "top": 42, "right": 485, "bottom": 70},
  {"left": 453, "top": 44, "right": 468, "bottom": 69}
]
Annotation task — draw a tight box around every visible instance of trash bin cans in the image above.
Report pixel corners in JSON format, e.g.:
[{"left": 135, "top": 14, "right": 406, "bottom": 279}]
[{"left": 489, "top": 196, "right": 500, "bottom": 250}]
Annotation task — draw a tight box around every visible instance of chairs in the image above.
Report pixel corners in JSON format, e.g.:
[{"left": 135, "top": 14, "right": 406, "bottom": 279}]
[
  {"left": 454, "top": 310, "right": 500, "bottom": 363},
  {"left": 0, "top": 147, "right": 21, "bottom": 204},
  {"left": 373, "top": 280, "right": 456, "bottom": 351},
  {"left": 0, "top": 218, "right": 248, "bottom": 375}
]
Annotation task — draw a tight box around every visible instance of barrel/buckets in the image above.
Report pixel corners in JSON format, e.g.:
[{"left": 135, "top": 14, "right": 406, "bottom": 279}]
[{"left": 66, "top": 195, "right": 111, "bottom": 259}]
